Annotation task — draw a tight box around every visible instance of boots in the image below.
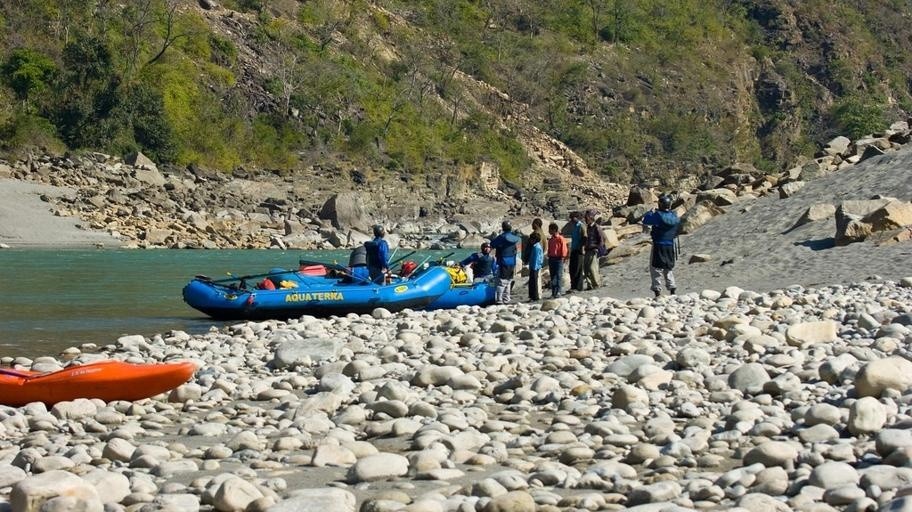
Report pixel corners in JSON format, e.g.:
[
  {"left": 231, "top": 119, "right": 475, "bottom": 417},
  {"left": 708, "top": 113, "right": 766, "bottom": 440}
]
[
  {"left": 671, "top": 289, "right": 675, "bottom": 294},
  {"left": 655, "top": 291, "right": 661, "bottom": 297}
]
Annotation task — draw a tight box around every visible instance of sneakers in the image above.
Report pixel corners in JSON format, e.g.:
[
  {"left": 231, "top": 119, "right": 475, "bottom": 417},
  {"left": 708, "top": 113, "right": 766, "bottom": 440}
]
[
  {"left": 526, "top": 284, "right": 601, "bottom": 303},
  {"left": 493, "top": 300, "right": 510, "bottom": 305}
]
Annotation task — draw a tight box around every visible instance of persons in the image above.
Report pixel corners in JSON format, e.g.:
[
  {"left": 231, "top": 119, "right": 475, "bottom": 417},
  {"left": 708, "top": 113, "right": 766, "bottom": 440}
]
[
  {"left": 642, "top": 196, "right": 680, "bottom": 297},
  {"left": 458, "top": 212, "right": 605, "bottom": 304},
  {"left": 366, "top": 226, "right": 389, "bottom": 283}
]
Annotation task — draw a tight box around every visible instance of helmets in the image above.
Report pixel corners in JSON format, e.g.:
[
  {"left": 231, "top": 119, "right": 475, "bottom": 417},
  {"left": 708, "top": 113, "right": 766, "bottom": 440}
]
[
  {"left": 374, "top": 225, "right": 384, "bottom": 236},
  {"left": 481, "top": 243, "right": 490, "bottom": 250},
  {"left": 502, "top": 221, "right": 512, "bottom": 231},
  {"left": 659, "top": 196, "right": 671, "bottom": 209}
]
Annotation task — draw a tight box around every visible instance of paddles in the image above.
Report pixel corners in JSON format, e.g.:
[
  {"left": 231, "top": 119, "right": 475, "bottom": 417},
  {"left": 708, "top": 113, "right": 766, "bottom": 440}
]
[
  {"left": 372, "top": 260, "right": 404, "bottom": 284},
  {"left": 452, "top": 282, "right": 489, "bottom": 288},
  {"left": 203, "top": 264, "right": 327, "bottom": 283}
]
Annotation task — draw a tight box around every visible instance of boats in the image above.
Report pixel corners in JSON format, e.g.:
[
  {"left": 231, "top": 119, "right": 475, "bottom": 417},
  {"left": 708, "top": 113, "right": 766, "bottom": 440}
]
[
  {"left": 0, "top": 356, "right": 198, "bottom": 409},
  {"left": 177, "top": 262, "right": 458, "bottom": 323},
  {"left": 395, "top": 269, "right": 503, "bottom": 311}
]
